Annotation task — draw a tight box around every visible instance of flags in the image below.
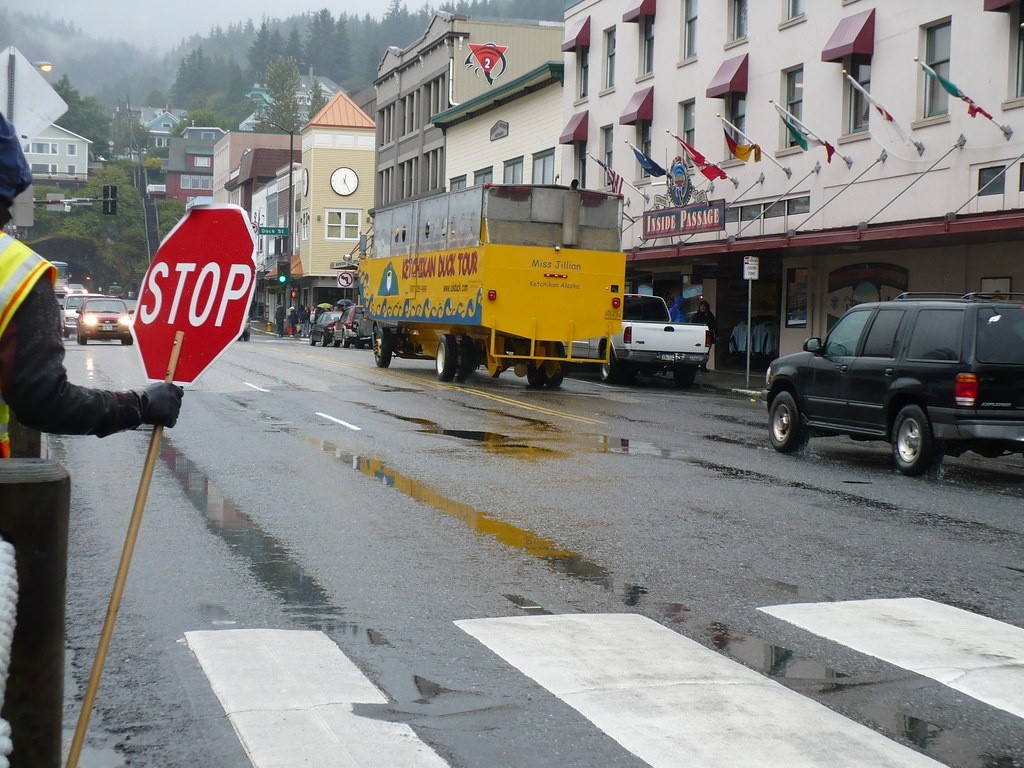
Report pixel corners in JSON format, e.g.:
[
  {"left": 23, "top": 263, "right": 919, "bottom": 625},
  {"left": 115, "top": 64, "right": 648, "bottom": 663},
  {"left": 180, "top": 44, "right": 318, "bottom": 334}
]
[
  {"left": 723, "top": 126, "right": 762, "bottom": 162},
  {"left": 597, "top": 159, "right": 623, "bottom": 193},
  {"left": 921, "top": 63, "right": 993, "bottom": 120},
  {"left": 781, "top": 115, "right": 835, "bottom": 163},
  {"left": 848, "top": 75, "right": 911, "bottom": 144},
  {"left": 674, "top": 135, "right": 727, "bottom": 181},
  {"left": 633, "top": 149, "right": 667, "bottom": 177}
]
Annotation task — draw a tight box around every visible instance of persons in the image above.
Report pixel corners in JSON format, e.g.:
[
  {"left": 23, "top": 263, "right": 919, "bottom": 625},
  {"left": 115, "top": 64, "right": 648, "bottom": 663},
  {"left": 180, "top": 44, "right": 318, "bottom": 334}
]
[
  {"left": 288, "top": 307, "right": 296, "bottom": 337},
  {"left": 670, "top": 297, "right": 687, "bottom": 322},
  {"left": 0, "top": 112, "right": 185, "bottom": 768},
  {"left": 692, "top": 300, "right": 720, "bottom": 373},
  {"left": 299, "top": 305, "right": 309, "bottom": 337},
  {"left": 310, "top": 304, "right": 316, "bottom": 329},
  {"left": 274, "top": 303, "right": 285, "bottom": 335}
]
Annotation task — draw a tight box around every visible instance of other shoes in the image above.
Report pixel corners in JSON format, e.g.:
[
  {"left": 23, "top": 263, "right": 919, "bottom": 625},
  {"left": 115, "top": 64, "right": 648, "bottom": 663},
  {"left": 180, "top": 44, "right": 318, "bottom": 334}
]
[{"left": 701, "top": 368, "right": 711, "bottom": 372}]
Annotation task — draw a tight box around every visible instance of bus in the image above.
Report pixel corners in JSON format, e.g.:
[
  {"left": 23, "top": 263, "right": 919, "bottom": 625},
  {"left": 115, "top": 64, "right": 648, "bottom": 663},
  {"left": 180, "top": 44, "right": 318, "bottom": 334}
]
[{"left": 52, "top": 262, "right": 72, "bottom": 289}]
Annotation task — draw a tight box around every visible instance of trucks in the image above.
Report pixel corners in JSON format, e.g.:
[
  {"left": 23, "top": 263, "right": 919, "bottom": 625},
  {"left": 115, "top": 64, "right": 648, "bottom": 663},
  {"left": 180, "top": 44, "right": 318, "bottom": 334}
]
[{"left": 343, "top": 186, "right": 627, "bottom": 392}]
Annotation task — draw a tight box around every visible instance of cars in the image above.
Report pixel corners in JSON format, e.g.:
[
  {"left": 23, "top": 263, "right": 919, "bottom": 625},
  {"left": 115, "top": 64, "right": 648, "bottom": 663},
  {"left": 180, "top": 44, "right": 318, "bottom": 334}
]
[
  {"left": 62, "top": 293, "right": 105, "bottom": 338},
  {"left": 310, "top": 311, "right": 344, "bottom": 349},
  {"left": 54, "top": 283, "right": 89, "bottom": 310},
  {"left": 76, "top": 298, "right": 135, "bottom": 346}
]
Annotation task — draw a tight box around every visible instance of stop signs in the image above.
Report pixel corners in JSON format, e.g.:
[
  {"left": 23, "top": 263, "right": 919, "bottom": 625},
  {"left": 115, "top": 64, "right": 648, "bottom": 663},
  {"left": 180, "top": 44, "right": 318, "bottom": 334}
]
[{"left": 126, "top": 202, "right": 259, "bottom": 387}]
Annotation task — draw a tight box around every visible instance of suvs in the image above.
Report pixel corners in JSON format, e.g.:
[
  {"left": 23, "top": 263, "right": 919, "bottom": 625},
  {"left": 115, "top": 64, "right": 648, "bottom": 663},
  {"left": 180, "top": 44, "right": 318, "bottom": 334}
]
[
  {"left": 762, "top": 290, "right": 1024, "bottom": 475},
  {"left": 332, "top": 306, "right": 374, "bottom": 351}
]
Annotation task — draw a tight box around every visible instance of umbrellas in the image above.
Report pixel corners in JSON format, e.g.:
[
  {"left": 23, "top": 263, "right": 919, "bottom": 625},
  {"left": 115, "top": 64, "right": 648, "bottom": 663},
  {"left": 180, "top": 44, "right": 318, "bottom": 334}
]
[
  {"left": 338, "top": 298, "right": 354, "bottom": 306},
  {"left": 317, "top": 303, "right": 333, "bottom": 309}
]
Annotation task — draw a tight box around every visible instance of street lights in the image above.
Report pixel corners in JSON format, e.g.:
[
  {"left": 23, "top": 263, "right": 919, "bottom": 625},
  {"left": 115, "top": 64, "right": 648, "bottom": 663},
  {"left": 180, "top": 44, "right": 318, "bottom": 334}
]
[{"left": 244, "top": 119, "right": 294, "bottom": 335}]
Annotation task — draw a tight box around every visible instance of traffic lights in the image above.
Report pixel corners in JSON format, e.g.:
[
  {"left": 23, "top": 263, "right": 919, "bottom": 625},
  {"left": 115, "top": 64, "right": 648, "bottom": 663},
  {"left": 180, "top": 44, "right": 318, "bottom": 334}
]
[
  {"left": 291, "top": 280, "right": 298, "bottom": 292},
  {"left": 276, "top": 260, "right": 291, "bottom": 285},
  {"left": 291, "top": 291, "right": 297, "bottom": 298}
]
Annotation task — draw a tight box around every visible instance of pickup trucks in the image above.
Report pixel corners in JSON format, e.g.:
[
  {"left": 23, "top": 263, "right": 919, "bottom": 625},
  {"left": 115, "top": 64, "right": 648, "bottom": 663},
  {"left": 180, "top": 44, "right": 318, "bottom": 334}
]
[{"left": 564, "top": 292, "right": 713, "bottom": 386}]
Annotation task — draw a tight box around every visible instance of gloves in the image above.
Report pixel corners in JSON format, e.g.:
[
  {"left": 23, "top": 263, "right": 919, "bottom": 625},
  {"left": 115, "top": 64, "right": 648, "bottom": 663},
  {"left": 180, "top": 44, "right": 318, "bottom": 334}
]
[{"left": 140, "top": 382, "right": 184, "bottom": 428}]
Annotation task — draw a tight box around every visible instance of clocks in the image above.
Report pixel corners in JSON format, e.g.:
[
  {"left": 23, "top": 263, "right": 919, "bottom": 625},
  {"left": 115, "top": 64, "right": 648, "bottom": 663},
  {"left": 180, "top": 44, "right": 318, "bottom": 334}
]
[
  {"left": 330, "top": 166, "right": 359, "bottom": 197},
  {"left": 302, "top": 169, "right": 308, "bottom": 196}
]
